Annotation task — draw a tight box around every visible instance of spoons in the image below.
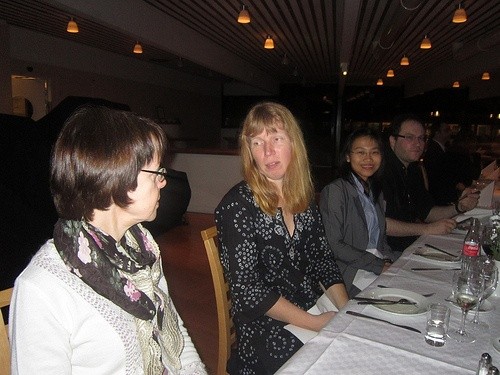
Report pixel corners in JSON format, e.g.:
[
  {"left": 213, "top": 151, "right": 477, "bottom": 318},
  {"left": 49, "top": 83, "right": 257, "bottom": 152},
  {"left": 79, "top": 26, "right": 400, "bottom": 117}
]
[{"left": 351, "top": 297, "right": 410, "bottom": 303}]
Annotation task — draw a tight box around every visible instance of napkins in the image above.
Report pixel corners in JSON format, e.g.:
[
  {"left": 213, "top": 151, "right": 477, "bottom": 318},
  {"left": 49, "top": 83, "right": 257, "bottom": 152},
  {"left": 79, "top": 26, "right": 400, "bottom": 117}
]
[
  {"left": 283, "top": 293, "right": 341, "bottom": 344},
  {"left": 456, "top": 207, "right": 493, "bottom": 223},
  {"left": 352, "top": 248, "right": 386, "bottom": 291}
]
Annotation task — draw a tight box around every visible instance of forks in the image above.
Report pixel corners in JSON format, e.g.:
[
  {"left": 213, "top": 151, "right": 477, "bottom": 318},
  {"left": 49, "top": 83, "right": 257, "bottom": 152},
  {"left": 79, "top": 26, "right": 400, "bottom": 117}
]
[{"left": 377, "top": 284, "right": 436, "bottom": 297}]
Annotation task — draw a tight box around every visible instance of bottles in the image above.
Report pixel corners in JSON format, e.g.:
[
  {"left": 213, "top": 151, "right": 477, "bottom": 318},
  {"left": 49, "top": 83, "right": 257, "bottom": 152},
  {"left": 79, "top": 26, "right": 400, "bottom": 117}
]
[
  {"left": 477, "top": 352, "right": 499, "bottom": 375},
  {"left": 462, "top": 217, "right": 481, "bottom": 271}
]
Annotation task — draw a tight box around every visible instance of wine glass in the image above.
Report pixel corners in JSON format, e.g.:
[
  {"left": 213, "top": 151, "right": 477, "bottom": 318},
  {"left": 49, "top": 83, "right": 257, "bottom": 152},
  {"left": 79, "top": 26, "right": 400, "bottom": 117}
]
[
  {"left": 446, "top": 270, "right": 476, "bottom": 344},
  {"left": 464, "top": 258, "right": 500, "bottom": 333}
]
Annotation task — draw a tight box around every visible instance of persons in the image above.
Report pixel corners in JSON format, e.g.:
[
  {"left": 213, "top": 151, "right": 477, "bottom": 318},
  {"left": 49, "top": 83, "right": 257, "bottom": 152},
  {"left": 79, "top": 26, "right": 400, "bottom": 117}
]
[
  {"left": 425, "top": 119, "right": 500, "bottom": 202},
  {"left": 7, "top": 105, "right": 210, "bottom": 375},
  {"left": 213, "top": 102, "right": 349, "bottom": 375},
  {"left": 371, "top": 115, "right": 481, "bottom": 259},
  {"left": 321, "top": 126, "right": 402, "bottom": 300}
]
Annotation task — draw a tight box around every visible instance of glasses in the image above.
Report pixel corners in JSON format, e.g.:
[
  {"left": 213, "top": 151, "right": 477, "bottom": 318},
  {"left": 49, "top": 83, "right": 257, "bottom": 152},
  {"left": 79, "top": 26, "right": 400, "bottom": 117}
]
[
  {"left": 351, "top": 149, "right": 383, "bottom": 157},
  {"left": 141, "top": 167, "right": 167, "bottom": 183},
  {"left": 396, "top": 134, "right": 428, "bottom": 143}
]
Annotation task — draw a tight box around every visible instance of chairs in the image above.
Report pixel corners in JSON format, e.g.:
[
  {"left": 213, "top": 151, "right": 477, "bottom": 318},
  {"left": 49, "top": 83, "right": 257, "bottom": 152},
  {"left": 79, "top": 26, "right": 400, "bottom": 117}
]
[
  {"left": 201, "top": 226, "right": 238, "bottom": 375},
  {"left": 0, "top": 288, "right": 14, "bottom": 375}
]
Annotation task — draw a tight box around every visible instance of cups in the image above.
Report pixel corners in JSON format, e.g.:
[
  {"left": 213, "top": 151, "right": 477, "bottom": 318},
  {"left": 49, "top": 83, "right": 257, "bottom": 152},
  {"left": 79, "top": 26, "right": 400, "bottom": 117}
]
[
  {"left": 482, "top": 219, "right": 500, "bottom": 261},
  {"left": 472, "top": 179, "right": 495, "bottom": 198},
  {"left": 424, "top": 303, "right": 450, "bottom": 348}
]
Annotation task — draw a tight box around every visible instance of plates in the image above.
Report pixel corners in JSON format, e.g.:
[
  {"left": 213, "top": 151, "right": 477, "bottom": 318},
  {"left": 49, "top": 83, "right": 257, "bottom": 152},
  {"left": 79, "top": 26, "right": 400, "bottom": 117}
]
[
  {"left": 478, "top": 259, "right": 500, "bottom": 286},
  {"left": 446, "top": 293, "right": 496, "bottom": 314},
  {"left": 413, "top": 246, "right": 462, "bottom": 263},
  {"left": 364, "top": 287, "right": 432, "bottom": 316},
  {"left": 464, "top": 209, "right": 493, "bottom": 218},
  {"left": 489, "top": 333, "right": 500, "bottom": 354}
]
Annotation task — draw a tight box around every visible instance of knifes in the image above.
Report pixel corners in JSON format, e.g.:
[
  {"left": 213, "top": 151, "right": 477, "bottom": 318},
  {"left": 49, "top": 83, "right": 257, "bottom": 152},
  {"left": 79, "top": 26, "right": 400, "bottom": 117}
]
[
  {"left": 411, "top": 267, "right": 461, "bottom": 271},
  {"left": 357, "top": 301, "right": 417, "bottom": 305},
  {"left": 424, "top": 244, "right": 458, "bottom": 258},
  {"left": 457, "top": 216, "right": 473, "bottom": 224},
  {"left": 346, "top": 311, "right": 421, "bottom": 334}
]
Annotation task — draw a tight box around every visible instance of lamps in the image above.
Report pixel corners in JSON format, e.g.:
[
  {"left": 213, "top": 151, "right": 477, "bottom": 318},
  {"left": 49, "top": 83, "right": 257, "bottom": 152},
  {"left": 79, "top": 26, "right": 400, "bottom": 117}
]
[
  {"left": 66, "top": 17, "right": 79, "bottom": 33},
  {"left": 452, "top": 2, "right": 467, "bottom": 23},
  {"left": 419, "top": 34, "right": 431, "bottom": 49},
  {"left": 376, "top": 55, "right": 490, "bottom": 88},
  {"left": 133, "top": 41, "right": 143, "bottom": 54},
  {"left": 237, "top": 5, "right": 250, "bottom": 24},
  {"left": 264, "top": 36, "right": 275, "bottom": 50}
]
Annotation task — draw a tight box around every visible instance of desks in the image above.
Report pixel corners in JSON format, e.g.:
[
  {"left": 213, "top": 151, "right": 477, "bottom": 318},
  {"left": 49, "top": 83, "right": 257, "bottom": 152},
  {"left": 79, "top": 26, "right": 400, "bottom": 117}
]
[{"left": 272, "top": 227, "right": 500, "bottom": 374}]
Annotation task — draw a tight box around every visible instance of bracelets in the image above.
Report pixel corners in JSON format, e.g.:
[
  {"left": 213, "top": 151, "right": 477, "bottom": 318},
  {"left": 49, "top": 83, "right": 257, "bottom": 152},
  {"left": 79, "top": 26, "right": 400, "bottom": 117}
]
[
  {"left": 455, "top": 201, "right": 462, "bottom": 213},
  {"left": 383, "top": 258, "right": 391, "bottom": 263}
]
